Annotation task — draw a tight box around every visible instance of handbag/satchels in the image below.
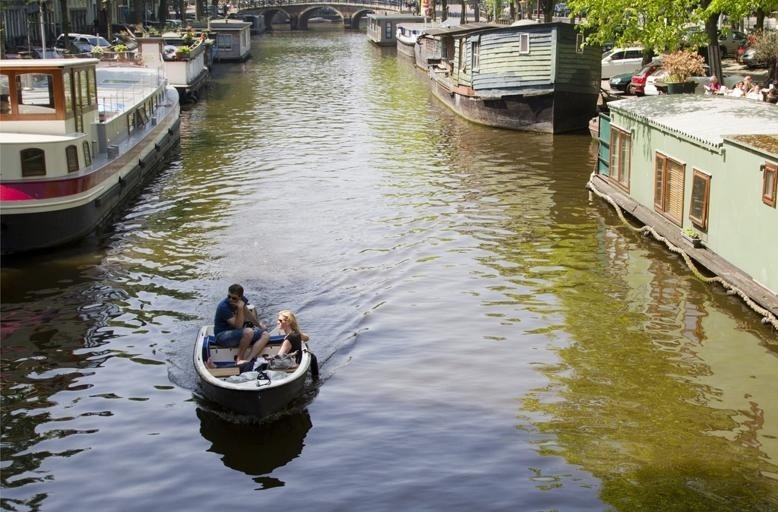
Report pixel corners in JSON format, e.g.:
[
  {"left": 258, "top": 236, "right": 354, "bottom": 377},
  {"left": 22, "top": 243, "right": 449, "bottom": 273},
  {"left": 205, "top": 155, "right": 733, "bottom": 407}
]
[
  {"left": 241, "top": 357, "right": 267, "bottom": 371},
  {"left": 269, "top": 353, "right": 297, "bottom": 369}
]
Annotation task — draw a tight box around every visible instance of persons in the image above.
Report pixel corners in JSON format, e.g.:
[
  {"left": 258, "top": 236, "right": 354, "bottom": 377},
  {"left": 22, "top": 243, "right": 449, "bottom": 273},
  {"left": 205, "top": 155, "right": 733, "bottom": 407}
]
[
  {"left": 214, "top": 283, "right": 272, "bottom": 366},
  {"left": 706, "top": 75, "right": 778, "bottom": 105},
  {"left": 265, "top": 310, "right": 309, "bottom": 361}
]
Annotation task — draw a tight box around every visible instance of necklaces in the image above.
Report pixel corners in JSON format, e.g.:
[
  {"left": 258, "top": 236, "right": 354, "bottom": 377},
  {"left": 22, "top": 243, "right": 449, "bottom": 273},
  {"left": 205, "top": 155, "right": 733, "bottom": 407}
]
[{"left": 283, "top": 331, "right": 292, "bottom": 340}]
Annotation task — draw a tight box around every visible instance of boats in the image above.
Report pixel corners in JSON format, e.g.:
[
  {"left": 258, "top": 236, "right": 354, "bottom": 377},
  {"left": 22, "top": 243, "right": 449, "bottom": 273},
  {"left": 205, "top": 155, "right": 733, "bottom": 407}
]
[
  {"left": 192, "top": 302, "right": 312, "bottom": 417},
  {"left": 0, "top": 67, "right": 184, "bottom": 254}
]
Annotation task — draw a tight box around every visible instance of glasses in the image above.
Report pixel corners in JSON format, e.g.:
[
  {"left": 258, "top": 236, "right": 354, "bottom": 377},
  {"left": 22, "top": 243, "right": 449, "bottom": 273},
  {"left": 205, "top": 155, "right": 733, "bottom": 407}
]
[{"left": 227, "top": 295, "right": 237, "bottom": 300}]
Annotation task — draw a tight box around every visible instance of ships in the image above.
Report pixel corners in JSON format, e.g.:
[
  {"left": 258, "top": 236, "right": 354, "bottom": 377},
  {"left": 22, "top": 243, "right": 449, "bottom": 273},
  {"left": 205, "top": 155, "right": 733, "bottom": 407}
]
[{"left": 427, "top": 33, "right": 601, "bottom": 135}]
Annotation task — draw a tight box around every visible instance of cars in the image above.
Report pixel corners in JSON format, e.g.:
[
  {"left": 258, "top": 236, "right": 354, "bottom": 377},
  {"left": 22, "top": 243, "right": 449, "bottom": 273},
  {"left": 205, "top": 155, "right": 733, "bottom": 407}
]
[
  {"left": 55, "top": 31, "right": 131, "bottom": 58},
  {"left": 600, "top": 46, "right": 745, "bottom": 96}
]
